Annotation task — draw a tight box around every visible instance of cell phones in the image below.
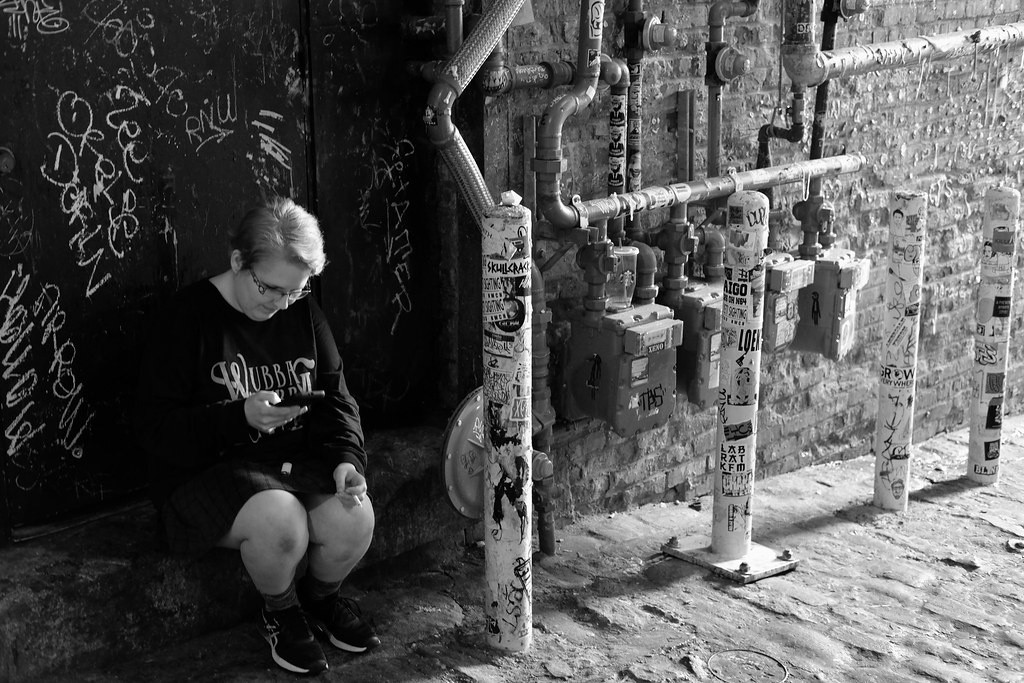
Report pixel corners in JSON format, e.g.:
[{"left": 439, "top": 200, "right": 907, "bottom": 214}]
[{"left": 274, "top": 390, "right": 325, "bottom": 408}]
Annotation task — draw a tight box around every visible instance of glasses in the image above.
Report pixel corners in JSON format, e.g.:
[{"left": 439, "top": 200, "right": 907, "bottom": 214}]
[{"left": 242, "top": 263, "right": 311, "bottom": 300}]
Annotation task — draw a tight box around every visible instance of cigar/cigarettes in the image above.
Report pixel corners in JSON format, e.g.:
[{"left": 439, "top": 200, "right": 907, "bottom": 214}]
[{"left": 352, "top": 494, "right": 362, "bottom": 508}]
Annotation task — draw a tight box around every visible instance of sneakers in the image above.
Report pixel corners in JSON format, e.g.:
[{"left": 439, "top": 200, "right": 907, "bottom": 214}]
[
  {"left": 297, "top": 591, "right": 381, "bottom": 653},
  {"left": 253, "top": 601, "right": 329, "bottom": 675}
]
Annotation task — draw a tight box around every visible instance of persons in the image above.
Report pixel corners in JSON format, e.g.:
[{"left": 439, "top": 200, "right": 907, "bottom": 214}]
[{"left": 153, "top": 198, "right": 382, "bottom": 672}]
